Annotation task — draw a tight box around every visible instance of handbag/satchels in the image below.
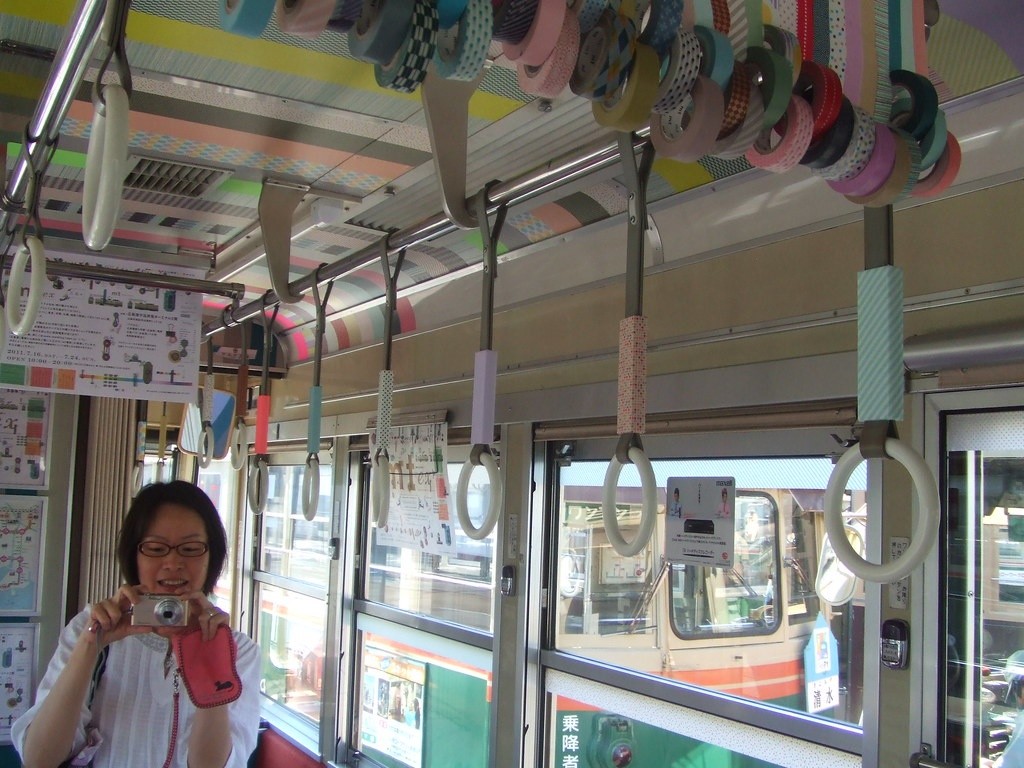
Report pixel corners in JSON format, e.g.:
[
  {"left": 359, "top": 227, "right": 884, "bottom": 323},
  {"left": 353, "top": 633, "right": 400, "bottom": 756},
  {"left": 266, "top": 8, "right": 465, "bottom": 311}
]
[{"left": 65, "top": 645, "right": 110, "bottom": 768}]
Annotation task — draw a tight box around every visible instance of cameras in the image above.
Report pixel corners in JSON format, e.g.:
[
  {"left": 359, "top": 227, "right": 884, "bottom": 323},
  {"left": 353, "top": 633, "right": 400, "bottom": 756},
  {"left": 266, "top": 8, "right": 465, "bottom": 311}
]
[{"left": 130, "top": 594, "right": 189, "bottom": 627}]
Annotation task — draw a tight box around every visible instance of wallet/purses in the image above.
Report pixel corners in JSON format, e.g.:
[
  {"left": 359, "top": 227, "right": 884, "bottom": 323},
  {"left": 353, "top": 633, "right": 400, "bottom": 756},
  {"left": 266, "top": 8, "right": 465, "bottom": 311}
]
[{"left": 173, "top": 623, "right": 242, "bottom": 710}]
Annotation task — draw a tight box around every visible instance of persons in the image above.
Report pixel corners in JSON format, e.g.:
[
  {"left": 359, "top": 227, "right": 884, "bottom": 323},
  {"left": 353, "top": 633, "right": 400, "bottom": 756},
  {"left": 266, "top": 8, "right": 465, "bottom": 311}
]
[
  {"left": 10, "top": 481, "right": 261, "bottom": 768},
  {"left": 715, "top": 488, "right": 730, "bottom": 518},
  {"left": 670, "top": 487, "right": 683, "bottom": 516}
]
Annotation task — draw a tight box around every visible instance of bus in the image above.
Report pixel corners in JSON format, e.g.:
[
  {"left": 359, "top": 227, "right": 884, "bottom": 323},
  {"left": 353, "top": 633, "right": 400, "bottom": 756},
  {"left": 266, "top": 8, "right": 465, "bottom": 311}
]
[{"left": 141, "top": 455, "right": 839, "bottom": 768}]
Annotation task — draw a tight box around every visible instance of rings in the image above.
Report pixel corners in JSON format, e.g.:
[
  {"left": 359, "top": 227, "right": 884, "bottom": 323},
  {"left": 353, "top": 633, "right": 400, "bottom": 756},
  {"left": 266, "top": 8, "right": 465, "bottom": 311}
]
[{"left": 207, "top": 611, "right": 213, "bottom": 617}]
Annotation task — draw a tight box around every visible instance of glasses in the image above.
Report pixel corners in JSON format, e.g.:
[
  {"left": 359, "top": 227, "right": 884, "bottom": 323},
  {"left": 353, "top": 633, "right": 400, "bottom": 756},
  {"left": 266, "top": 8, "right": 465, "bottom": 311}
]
[{"left": 137, "top": 540, "right": 210, "bottom": 557}]
